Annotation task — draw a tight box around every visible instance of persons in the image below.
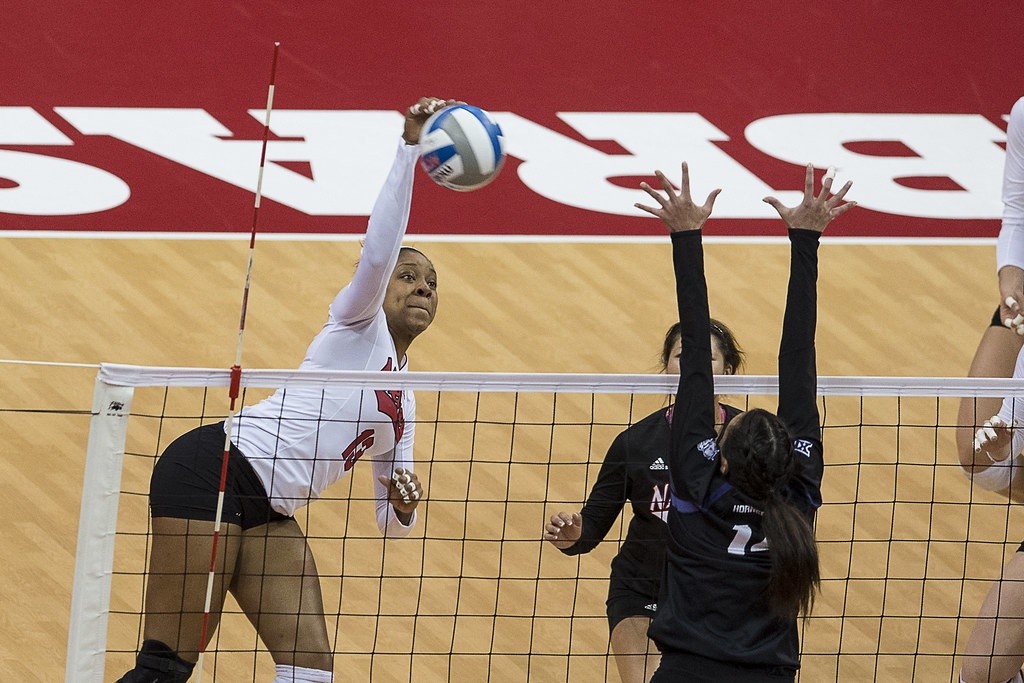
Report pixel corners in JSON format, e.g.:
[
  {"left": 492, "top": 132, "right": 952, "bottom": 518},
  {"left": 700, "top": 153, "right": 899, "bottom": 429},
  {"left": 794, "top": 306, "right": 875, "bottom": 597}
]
[
  {"left": 959, "top": 97, "right": 1024, "bottom": 683},
  {"left": 113, "top": 96, "right": 469, "bottom": 682},
  {"left": 634, "top": 162, "right": 857, "bottom": 683},
  {"left": 541, "top": 319, "right": 757, "bottom": 683}
]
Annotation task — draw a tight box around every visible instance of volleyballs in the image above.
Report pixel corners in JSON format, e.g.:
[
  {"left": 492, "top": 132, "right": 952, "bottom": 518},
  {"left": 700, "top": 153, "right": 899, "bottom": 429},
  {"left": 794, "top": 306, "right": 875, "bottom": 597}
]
[{"left": 417, "top": 101, "right": 508, "bottom": 193}]
